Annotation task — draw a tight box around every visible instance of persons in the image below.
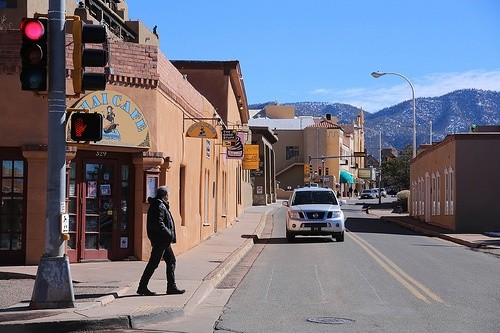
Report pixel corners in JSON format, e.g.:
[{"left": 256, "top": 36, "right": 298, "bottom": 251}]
[
  {"left": 349, "top": 186, "right": 353, "bottom": 197},
  {"left": 354, "top": 188, "right": 358, "bottom": 196},
  {"left": 137, "top": 185, "right": 186, "bottom": 296}
]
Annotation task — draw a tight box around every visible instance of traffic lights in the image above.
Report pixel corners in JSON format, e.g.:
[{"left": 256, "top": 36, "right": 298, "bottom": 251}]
[
  {"left": 73, "top": 20, "right": 107, "bottom": 94},
  {"left": 19, "top": 15, "right": 49, "bottom": 92},
  {"left": 71, "top": 113, "right": 103, "bottom": 141}
]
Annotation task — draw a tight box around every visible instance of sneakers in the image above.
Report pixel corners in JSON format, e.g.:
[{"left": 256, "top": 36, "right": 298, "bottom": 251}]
[
  {"left": 165, "top": 288, "right": 186, "bottom": 295},
  {"left": 136, "top": 286, "right": 157, "bottom": 296}
]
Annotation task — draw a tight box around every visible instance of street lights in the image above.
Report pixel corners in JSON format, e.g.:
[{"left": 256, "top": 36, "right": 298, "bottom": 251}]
[{"left": 371, "top": 70, "right": 417, "bottom": 158}]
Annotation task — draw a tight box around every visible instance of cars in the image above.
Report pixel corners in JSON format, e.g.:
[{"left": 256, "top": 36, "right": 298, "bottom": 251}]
[{"left": 361, "top": 187, "right": 387, "bottom": 199}]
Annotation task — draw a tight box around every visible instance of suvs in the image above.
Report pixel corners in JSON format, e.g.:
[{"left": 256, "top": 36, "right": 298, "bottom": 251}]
[{"left": 283, "top": 187, "right": 345, "bottom": 242}]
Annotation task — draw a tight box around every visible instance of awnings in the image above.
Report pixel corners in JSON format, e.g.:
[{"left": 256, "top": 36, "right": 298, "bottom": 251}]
[{"left": 340, "top": 169, "right": 354, "bottom": 183}]
